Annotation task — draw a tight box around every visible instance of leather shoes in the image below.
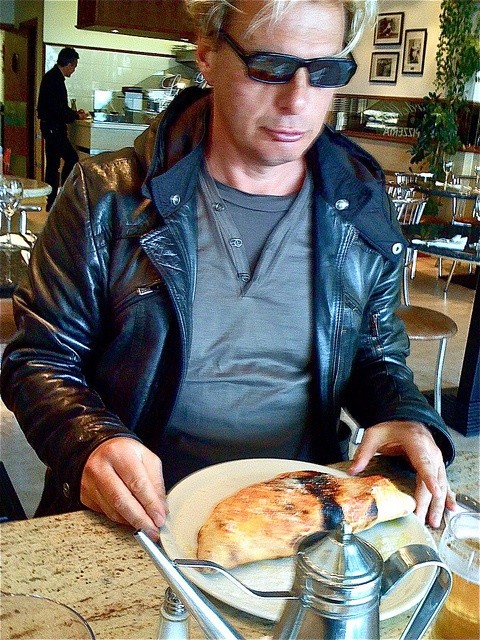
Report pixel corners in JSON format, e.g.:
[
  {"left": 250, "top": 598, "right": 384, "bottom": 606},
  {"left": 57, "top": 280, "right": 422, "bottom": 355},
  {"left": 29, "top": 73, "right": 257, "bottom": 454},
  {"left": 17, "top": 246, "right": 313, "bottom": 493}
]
[{"left": 45, "top": 201, "right": 54, "bottom": 211}]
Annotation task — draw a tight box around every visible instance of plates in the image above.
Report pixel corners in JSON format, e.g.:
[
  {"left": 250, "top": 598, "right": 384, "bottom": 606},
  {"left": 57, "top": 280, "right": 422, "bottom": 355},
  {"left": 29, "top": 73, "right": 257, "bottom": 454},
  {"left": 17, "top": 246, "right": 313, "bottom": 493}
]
[{"left": 156, "top": 456, "right": 440, "bottom": 623}]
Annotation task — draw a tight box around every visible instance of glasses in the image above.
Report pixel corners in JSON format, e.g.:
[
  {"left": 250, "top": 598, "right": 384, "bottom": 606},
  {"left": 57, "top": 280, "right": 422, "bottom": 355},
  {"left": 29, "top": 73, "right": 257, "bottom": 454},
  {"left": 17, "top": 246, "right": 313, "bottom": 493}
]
[{"left": 217, "top": 28, "right": 358, "bottom": 87}]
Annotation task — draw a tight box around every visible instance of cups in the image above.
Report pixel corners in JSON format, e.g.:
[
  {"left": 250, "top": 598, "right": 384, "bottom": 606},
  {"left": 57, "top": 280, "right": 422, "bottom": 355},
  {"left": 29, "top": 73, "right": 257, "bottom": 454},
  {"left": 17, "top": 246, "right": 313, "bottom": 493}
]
[{"left": 441, "top": 509, "right": 480, "bottom": 640}]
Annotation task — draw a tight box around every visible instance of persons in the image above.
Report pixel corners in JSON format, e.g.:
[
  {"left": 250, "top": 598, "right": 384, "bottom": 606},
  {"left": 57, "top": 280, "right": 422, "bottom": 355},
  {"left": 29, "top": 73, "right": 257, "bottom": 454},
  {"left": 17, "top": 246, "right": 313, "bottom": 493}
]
[
  {"left": 0, "top": 0, "right": 457, "bottom": 546},
  {"left": 37, "top": 44, "right": 89, "bottom": 214}
]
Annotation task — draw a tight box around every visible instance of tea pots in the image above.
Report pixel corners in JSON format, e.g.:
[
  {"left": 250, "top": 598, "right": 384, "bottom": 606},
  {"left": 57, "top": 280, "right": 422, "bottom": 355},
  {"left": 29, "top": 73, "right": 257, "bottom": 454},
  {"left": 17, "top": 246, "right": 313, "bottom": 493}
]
[{"left": 133, "top": 521, "right": 451, "bottom": 640}]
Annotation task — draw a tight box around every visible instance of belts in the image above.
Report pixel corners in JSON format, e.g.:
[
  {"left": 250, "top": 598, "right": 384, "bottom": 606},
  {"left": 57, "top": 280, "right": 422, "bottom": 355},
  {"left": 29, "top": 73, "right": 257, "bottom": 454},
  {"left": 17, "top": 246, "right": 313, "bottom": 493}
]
[{"left": 39, "top": 117, "right": 51, "bottom": 125}]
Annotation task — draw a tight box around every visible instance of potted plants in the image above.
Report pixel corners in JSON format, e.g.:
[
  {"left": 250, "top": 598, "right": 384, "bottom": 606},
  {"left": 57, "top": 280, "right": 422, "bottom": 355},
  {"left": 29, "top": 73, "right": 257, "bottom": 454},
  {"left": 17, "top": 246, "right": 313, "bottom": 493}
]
[{"left": 398, "top": 0, "right": 480, "bottom": 225}]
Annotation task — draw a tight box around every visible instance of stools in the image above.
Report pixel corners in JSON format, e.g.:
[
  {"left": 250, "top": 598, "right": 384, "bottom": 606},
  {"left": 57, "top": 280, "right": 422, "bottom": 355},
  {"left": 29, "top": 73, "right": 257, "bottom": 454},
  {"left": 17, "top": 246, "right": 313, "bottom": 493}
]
[{"left": 393, "top": 306, "right": 458, "bottom": 416}]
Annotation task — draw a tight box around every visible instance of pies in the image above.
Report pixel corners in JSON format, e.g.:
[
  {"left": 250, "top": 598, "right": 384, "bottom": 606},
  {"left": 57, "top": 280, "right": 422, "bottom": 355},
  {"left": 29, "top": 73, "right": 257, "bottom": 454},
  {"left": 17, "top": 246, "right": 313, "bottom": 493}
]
[{"left": 197, "top": 471, "right": 416, "bottom": 575}]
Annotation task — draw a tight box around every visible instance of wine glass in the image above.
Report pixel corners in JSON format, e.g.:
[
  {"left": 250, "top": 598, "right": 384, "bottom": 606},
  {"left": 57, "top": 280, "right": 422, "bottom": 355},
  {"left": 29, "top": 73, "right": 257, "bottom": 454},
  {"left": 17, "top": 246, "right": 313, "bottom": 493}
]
[
  {"left": 2, "top": 249, "right": 19, "bottom": 288},
  {"left": 438, "top": 155, "right": 456, "bottom": 191},
  {"left": 0, "top": 179, "right": 24, "bottom": 250}
]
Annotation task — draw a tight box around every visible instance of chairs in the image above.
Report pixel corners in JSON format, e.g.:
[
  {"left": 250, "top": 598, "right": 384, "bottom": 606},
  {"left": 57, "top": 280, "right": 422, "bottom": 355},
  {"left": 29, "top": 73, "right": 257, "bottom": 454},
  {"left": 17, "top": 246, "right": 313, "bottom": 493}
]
[
  {"left": 0, "top": 204, "right": 42, "bottom": 235},
  {"left": 383, "top": 173, "right": 427, "bottom": 226}
]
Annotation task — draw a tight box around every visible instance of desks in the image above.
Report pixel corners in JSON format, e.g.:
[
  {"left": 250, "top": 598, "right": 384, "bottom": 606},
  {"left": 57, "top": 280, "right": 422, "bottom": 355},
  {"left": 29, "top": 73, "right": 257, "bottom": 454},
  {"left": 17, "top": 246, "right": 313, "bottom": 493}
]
[
  {"left": 399, "top": 220, "right": 480, "bottom": 291},
  {"left": 0, "top": 175, "right": 52, "bottom": 199},
  {"left": 410, "top": 181, "right": 479, "bottom": 221}
]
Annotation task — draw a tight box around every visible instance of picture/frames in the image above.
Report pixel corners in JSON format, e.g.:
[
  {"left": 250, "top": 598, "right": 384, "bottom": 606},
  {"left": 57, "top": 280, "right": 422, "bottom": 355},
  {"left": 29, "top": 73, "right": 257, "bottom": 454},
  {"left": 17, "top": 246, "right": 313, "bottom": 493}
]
[
  {"left": 368, "top": 51, "right": 400, "bottom": 83},
  {"left": 373, "top": 13, "right": 404, "bottom": 45},
  {"left": 401, "top": 27, "right": 427, "bottom": 74}
]
